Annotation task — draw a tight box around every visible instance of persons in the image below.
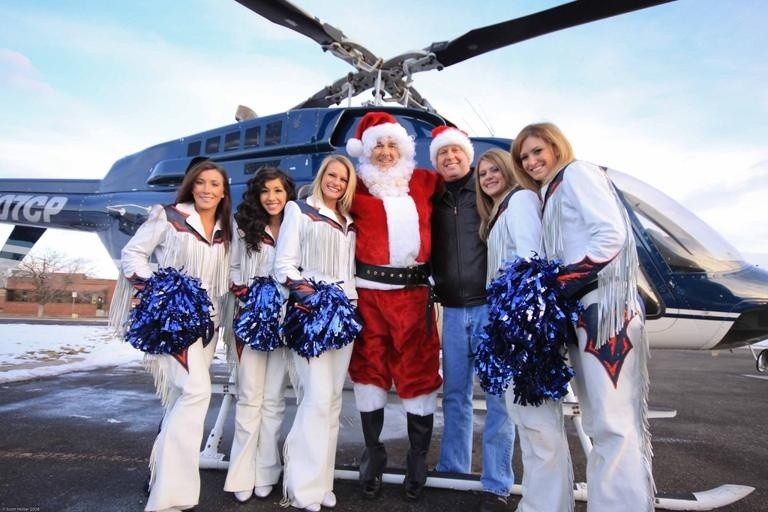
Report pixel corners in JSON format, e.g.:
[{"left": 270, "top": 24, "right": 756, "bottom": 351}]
[
  {"left": 118, "top": 161, "right": 233, "bottom": 512},
  {"left": 428, "top": 124, "right": 516, "bottom": 512},
  {"left": 343, "top": 111, "right": 442, "bottom": 503},
  {"left": 508, "top": 120, "right": 658, "bottom": 512},
  {"left": 222, "top": 168, "right": 298, "bottom": 502},
  {"left": 272, "top": 154, "right": 361, "bottom": 512},
  {"left": 475, "top": 147, "right": 577, "bottom": 512}
]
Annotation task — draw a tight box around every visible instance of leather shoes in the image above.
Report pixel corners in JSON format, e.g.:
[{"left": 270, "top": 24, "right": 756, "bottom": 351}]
[{"left": 232, "top": 487, "right": 337, "bottom": 512}]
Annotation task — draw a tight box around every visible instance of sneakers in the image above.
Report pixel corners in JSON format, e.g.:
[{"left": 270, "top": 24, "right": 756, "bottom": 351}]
[{"left": 476, "top": 491, "right": 508, "bottom": 510}]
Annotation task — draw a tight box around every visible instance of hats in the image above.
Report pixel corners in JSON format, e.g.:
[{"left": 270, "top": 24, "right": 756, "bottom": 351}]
[
  {"left": 346, "top": 109, "right": 410, "bottom": 157},
  {"left": 428, "top": 126, "right": 475, "bottom": 169}
]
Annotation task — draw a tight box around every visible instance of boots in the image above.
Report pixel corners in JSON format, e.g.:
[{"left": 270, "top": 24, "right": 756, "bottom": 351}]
[
  {"left": 354, "top": 408, "right": 387, "bottom": 500},
  {"left": 401, "top": 411, "right": 434, "bottom": 500}
]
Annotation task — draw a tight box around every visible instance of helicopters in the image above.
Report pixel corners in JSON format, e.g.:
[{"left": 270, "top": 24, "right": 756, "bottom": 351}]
[{"left": 1, "top": 0, "right": 767, "bottom": 511}]
